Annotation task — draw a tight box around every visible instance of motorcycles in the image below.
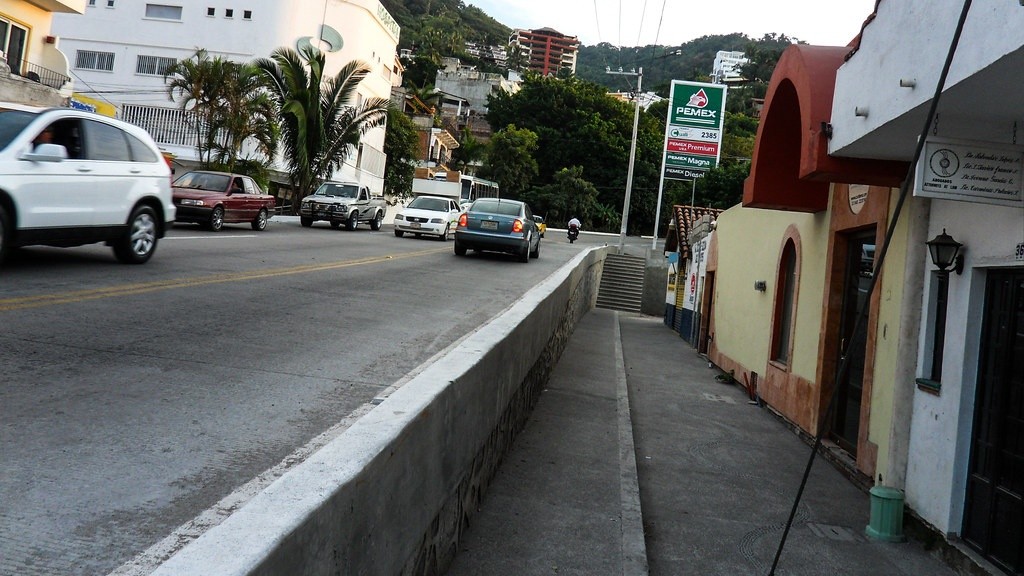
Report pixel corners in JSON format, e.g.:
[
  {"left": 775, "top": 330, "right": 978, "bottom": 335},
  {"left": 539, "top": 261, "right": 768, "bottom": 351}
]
[{"left": 567, "top": 223, "right": 581, "bottom": 243}]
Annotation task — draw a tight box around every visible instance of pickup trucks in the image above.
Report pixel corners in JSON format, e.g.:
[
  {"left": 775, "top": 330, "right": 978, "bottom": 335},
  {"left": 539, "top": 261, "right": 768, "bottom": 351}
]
[{"left": 300, "top": 181, "right": 388, "bottom": 231}]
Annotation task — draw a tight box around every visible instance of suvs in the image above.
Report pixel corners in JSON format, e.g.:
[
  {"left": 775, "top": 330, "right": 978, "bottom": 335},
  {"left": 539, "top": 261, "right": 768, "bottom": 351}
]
[{"left": 0, "top": 103, "right": 176, "bottom": 269}]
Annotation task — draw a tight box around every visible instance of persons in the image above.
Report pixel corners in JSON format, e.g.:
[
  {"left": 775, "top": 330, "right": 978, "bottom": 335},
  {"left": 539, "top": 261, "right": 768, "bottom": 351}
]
[
  {"left": 39, "top": 123, "right": 57, "bottom": 144},
  {"left": 566, "top": 214, "right": 582, "bottom": 238},
  {"left": 231, "top": 181, "right": 244, "bottom": 193}
]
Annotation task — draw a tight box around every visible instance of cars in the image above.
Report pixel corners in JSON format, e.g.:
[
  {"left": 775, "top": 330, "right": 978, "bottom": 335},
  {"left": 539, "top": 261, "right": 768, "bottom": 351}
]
[
  {"left": 393, "top": 196, "right": 464, "bottom": 240},
  {"left": 532, "top": 216, "right": 547, "bottom": 239},
  {"left": 170, "top": 171, "right": 276, "bottom": 232},
  {"left": 458, "top": 197, "right": 541, "bottom": 263}
]
